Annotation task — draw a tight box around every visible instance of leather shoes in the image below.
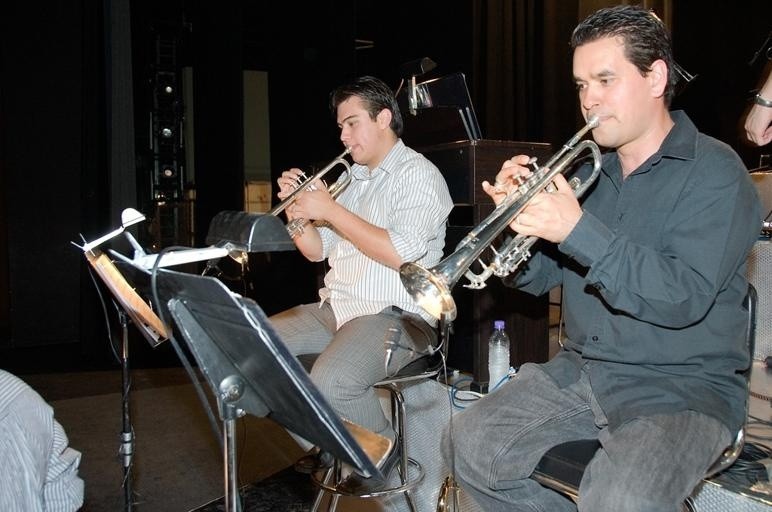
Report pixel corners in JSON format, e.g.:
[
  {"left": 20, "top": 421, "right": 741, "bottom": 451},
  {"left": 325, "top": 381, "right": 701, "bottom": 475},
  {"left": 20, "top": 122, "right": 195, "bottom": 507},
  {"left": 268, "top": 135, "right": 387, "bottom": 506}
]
[{"left": 292, "top": 436, "right": 407, "bottom": 496}]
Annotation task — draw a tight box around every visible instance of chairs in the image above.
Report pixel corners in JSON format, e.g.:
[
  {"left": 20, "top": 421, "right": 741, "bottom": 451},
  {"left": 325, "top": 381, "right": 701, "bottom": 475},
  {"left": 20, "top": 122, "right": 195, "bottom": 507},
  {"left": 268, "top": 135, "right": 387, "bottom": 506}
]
[
  {"left": 527, "top": 282, "right": 759, "bottom": 511},
  {"left": 308, "top": 324, "right": 450, "bottom": 511}
]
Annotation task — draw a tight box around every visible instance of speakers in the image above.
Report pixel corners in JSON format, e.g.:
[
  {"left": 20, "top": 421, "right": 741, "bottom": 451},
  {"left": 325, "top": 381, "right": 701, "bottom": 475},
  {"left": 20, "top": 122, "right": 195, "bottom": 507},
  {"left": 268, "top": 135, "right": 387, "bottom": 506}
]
[{"left": 405, "top": 137, "right": 552, "bottom": 383}]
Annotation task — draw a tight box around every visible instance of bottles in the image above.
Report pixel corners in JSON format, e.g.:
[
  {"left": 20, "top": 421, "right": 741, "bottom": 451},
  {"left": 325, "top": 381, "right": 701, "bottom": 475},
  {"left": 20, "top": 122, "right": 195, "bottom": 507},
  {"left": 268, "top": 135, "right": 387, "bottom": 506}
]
[{"left": 487, "top": 320, "right": 511, "bottom": 393}]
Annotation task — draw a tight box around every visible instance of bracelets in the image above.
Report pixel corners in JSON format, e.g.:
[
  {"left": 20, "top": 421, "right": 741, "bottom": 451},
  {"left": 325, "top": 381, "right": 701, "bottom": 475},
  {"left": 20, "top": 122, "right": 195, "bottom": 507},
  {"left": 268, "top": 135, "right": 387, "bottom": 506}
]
[{"left": 753, "top": 93, "right": 772, "bottom": 108}]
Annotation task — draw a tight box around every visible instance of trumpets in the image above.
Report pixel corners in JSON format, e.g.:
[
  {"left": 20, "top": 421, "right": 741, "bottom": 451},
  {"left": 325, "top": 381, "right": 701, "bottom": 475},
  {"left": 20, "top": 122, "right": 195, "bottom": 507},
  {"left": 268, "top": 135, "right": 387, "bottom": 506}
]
[
  {"left": 225, "top": 146, "right": 352, "bottom": 265},
  {"left": 400, "top": 113, "right": 602, "bottom": 324}
]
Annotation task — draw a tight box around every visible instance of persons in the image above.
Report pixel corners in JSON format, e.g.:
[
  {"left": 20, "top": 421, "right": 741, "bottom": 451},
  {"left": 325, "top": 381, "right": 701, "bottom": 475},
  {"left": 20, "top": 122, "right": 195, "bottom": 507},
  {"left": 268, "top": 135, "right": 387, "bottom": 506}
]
[
  {"left": 266, "top": 74, "right": 454, "bottom": 480},
  {"left": 744, "top": 73, "right": 772, "bottom": 146},
  {"left": 0, "top": 368, "right": 85, "bottom": 511},
  {"left": 440, "top": 5, "right": 763, "bottom": 511}
]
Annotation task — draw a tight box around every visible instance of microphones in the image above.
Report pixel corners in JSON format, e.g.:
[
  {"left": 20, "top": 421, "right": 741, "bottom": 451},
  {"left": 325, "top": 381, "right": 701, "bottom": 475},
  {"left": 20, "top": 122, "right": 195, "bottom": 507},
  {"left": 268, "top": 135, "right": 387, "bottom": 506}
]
[
  {"left": 202, "top": 258, "right": 219, "bottom": 276},
  {"left": 383, "top": 326, "right": 402, "bottom": 367}
]
